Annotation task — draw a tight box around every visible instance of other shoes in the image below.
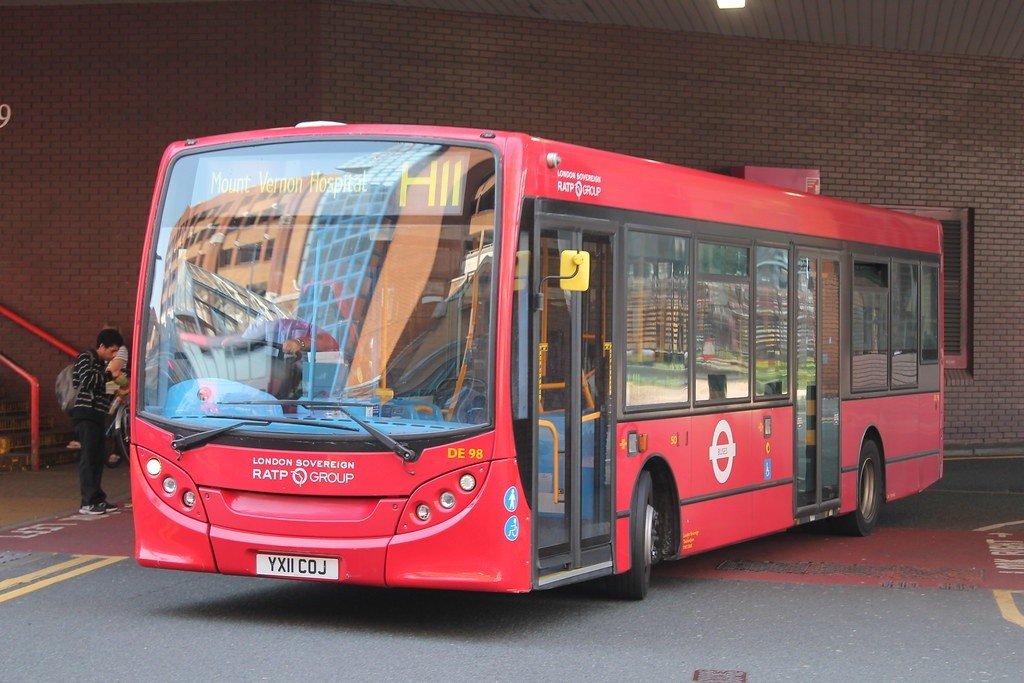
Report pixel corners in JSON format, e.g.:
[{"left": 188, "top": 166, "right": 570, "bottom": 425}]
[
  {"left": 106, "top": 453, "right": 123, "bottom": 466},
  {"left": 65, "top": 438, "right": 82, "bottom": 450}
]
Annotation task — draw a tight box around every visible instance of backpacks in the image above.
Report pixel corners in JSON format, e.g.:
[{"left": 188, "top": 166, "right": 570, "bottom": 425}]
[{"left": 53, "top": 350, "right": 95, "bottom": 414}]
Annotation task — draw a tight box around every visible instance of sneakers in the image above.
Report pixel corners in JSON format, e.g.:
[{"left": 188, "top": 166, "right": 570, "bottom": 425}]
[
  {"left": 96, "top": 500, "right": 120, "bottom": 513},
  {"left": 77, "top": 502, "right": 106, "bottom": 516}
]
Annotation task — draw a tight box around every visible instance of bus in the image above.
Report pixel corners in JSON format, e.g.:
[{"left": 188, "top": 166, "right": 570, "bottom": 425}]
[{"left": 128, "top": 121, "right": 946, "bottom": 600}]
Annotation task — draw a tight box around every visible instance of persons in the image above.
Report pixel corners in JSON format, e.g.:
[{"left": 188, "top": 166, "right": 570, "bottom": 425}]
[
  {"left": 175, "top": 267, "right": 340, "bottom": 414},
  {"left": 65, "top": 329, "right": 128, "bottom": 515}
]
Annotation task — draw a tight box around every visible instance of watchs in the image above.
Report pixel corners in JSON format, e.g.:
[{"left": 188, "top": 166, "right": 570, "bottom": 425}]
[{"left": 296, "top": 338, "right": 305, "bottom": 349}]
